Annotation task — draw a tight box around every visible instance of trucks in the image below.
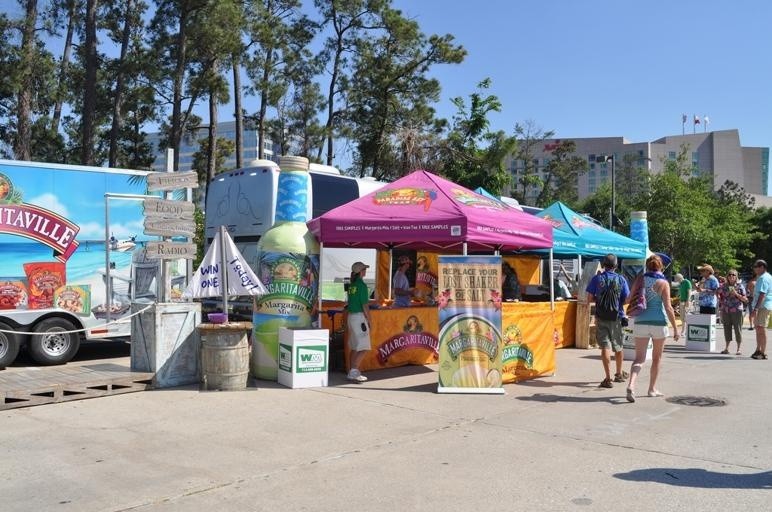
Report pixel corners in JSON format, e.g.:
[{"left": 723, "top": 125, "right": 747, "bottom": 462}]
[{"left": 1, "top": 159, "right": 186, "bottom": 369}]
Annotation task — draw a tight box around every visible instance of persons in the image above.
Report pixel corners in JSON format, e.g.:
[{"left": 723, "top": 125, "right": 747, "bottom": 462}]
[
  {"left": 586, "top": 254, "right": 630, "bottom": 387},
  {"left": 538, "top": 276, "right": 572, "bottom": 300},
  {"left": 345, "top": 262, "right": 373, "bottom": 381},
  {"left": 501, "top": 262, "right": 524, "bottom": 300},
  {"left": 626, "top": 255, "right": 679, "bottom": 402},
  {"left": 675, "top": 260, "right": 772, "bottom": 360},
  {"left": 393, "top": 254, "right": 416, "bottom": 306}
]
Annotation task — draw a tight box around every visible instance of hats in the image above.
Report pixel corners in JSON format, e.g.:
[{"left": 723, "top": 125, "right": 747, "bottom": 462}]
[
  {"left": 352, "top": 262, "right": 369, "bottom": 273},
  {"left": 398, "top": 255, "right": 413, "bottom": 265},
  {"left": 675, "top": 274, "right": 683, "bottom": 281},
  {"left": 703, "top": 265, "right": 714, "bottom": 274},
  {"left": 715, "top": 269, "right": 720, "bottom": 273}
]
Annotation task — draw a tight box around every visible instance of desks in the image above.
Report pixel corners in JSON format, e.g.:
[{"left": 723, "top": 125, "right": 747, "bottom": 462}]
[{"left": 322, "top": 299, "right": 577, "bottom": 373}]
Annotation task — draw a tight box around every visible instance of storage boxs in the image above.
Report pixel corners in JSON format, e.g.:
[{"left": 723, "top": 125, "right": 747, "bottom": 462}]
[
  {"left": 686, "top": 314, "right": 717, "bottom": 351},
  {"left": 623, "top": 318, "right": 654, "bottom": 361},
  {"left": 278, "top": 327, "right": 330, "bottom": 389}
]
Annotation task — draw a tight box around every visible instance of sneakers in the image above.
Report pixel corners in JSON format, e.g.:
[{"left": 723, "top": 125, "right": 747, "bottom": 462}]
[
  {"left": 754, "top": 353, "right": 769, "bottom": 359},
  {"left": 347, "top": 369, "right": 367, "bottom": 382},
  {"left": 601, "top": 378, "right": 613, "bottom": 388},
  {"left": 752, "top": 350, "right": 761, "bottom": 358},
  {"left": 613, "top": 370, "right": 630, "bottom": 383},
  {"left": 736, "top": 350, "right": 742, "bottom": 355},
  {"left": 721, "top": 350, "right": 730, "bottom": 354}
]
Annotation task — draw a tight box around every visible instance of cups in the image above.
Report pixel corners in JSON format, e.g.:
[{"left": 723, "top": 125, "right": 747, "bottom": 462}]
[{"left": 728, "top": 286, "right": 734, "bottom": 293}]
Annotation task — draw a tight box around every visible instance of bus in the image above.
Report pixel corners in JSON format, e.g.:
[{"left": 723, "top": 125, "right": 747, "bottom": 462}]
[{"left": 204, "top": 158, "right": 524, "bottom": 311}]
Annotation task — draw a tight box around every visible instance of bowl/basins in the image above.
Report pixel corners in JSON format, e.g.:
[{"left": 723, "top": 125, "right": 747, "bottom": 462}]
[{"left": 205, "top": 313, "right": 227, "bottom": 324}]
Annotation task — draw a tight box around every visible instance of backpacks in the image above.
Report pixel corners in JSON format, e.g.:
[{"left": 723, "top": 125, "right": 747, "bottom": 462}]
[{"left": 598, "top": 273, "right": 620, "bottom": 315}]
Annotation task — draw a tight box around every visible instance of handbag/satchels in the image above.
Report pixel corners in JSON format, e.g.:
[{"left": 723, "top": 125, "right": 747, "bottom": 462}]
[{"left": 626, "top": 275, "right": 647, "bottom": 317}]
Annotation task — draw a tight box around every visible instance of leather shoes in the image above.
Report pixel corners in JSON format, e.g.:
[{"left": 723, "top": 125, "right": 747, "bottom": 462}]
[
  {"left": 626, "top": 387, "right": 636, "bottom": 402},
  {"left": 648, "top": 390, "right": 664, "bottom": 397}
]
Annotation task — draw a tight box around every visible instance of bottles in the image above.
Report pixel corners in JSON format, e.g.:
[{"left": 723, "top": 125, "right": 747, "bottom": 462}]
[
  {"left": 619, "top": 210, "right": 655, "bottom": 296},
  {"left": 247, "top": 153, "right": 323, "bottom": 383}
]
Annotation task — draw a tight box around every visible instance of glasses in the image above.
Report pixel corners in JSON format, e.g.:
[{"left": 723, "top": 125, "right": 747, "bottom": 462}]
[{"left": 729, "top": 274, "right": 736, "bottom": 276}]
